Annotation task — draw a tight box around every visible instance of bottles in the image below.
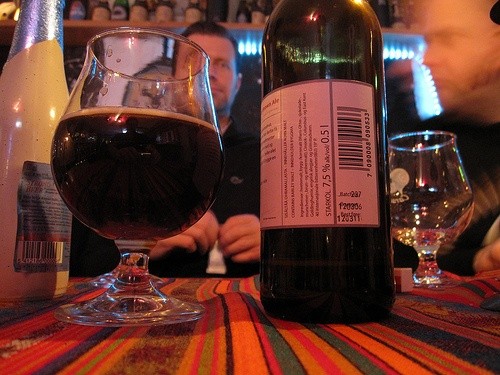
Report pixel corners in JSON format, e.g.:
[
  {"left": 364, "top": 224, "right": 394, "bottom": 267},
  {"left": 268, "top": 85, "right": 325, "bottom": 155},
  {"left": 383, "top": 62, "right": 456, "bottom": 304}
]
[
  {"left": 261, "top": 0, "right": 393, "bottom": 319},
  {"left": 0, "top": 0, "right": 72, "bottom": 306},
  {"left": 72, "top": 0, "right": 260, "bottom": 22}
]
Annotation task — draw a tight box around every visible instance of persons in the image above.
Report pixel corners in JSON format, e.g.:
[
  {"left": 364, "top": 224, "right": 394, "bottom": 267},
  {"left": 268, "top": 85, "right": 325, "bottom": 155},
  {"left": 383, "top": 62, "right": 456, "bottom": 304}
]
[
  {"left": 71, "top": 19, "right": 261, "bottom": 279},
  {"left": 392, "top": 0, "right": 500, "bottom": 278}
]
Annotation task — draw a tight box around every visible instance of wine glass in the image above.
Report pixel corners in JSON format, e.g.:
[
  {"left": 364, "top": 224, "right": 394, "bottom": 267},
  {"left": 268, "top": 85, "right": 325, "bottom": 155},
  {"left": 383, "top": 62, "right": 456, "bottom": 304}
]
[
  {"left": 387, "top": 131, "right": 474, "bottom": 291},
  {"left": 88, "top": 267, "right": 168, "bottom": 288},
  {"left": 51, "top": 30, "right": 224, "bottom": 326}
]
